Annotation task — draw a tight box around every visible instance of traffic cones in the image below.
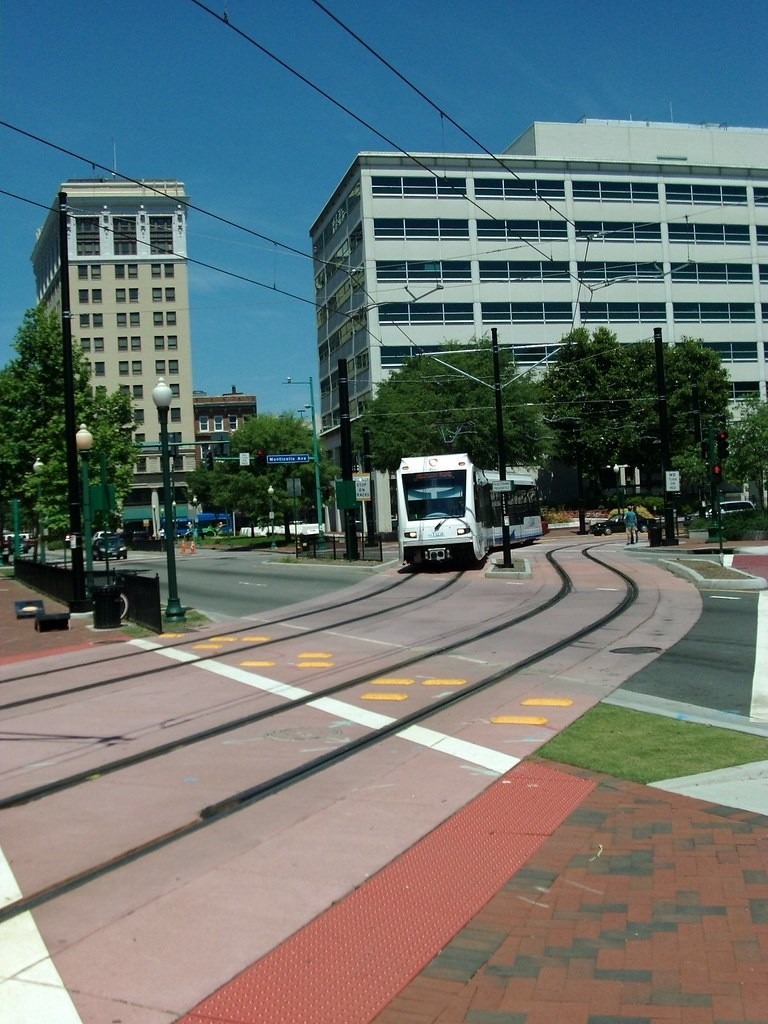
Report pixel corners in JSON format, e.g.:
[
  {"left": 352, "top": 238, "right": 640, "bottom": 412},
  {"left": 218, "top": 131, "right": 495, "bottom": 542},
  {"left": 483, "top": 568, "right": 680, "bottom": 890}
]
[
  {"left": 181, "top": 540, "right": 186, "bottom": 554},
  {"left": 190, "top": 541, "right": 197, "bottom": 554}
]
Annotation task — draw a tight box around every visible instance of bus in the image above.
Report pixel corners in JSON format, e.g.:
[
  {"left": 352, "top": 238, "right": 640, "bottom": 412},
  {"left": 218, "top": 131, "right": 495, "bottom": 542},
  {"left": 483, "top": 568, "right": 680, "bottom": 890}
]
[{"left": 396, "top": 452, "right": 545, "bottom": 567}]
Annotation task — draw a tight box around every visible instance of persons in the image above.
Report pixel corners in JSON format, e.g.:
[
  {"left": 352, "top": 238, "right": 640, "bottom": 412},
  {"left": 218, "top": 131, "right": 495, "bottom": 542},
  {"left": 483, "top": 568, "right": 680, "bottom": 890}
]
[{"left": 624, "top": 506, "right": 637, "bottom": 545}]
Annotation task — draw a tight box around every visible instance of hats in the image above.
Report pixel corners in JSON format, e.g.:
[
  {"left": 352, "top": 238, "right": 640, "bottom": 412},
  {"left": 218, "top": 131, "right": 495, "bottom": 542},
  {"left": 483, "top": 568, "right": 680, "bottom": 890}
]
[{"left": 627, "top": 506, "right": 633, "bottom": 509}]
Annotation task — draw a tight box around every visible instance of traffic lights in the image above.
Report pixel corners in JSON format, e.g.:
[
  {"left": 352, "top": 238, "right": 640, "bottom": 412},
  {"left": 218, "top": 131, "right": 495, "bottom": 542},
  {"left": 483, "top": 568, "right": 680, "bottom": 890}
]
[
  {"left": 718, "top": 432, "right": 729, "bottom": 459},
  {"left": 713, "top": 465, "right": 722, "bottom": 484}
]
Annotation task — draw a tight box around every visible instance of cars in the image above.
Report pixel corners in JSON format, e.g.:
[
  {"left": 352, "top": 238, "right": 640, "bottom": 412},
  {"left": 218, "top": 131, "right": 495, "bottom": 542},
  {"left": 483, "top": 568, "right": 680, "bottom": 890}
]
[
  {"left": 2, "top": 531, "right": 128, "bottom": 561},
  {"left": 589, "top": 513, "right": 656, "bottom": 536}
]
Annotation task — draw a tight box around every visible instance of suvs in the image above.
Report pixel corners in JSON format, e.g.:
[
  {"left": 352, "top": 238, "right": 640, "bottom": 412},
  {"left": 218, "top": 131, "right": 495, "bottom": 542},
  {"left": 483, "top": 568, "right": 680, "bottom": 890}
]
[{"left": 683, "top": 500, "right": 756, "bottom": 526}]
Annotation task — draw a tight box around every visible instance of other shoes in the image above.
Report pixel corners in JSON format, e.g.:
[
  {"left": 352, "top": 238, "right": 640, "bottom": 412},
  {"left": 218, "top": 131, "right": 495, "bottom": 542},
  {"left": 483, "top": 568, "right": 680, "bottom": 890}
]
[
  {"left": 633, "top": 542, "right": 636, "bottom": 543},
  {"left": 626, "top": 543, "right": 631, "bottom": 545}
]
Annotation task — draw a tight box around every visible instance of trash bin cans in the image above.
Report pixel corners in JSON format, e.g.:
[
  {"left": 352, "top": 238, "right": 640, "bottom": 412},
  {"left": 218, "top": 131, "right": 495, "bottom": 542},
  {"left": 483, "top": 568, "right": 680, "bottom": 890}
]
[
  {"left": 648, "top": 527, "right": 662, "bottom": 547},
  {"left": 91, "top": 585, "right": 121, "bottom": 628}
]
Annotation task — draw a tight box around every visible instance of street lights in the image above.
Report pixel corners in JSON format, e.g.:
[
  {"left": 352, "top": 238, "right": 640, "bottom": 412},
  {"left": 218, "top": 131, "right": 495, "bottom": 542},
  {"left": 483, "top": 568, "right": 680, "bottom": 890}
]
[
  {"left": 151, "top": 376, "right": 187, "bottom": 623},
  {"left": 32, "top": 458, "right": 48, "bottom": 566},
  {"left": 268, "top": 485, "right": 276, "bottom": 549},
  {"left": 151, "top": 504, "right": 158, "bottom": 540},
  {"left": 75, "top": 423, "right": 97, "bottom": 590},
  {"left": 192, "top": 497, "right": 197, "bottom": 541},
  {"left": 172, "top": 500, "right": 178, "bottom": 544}
]
